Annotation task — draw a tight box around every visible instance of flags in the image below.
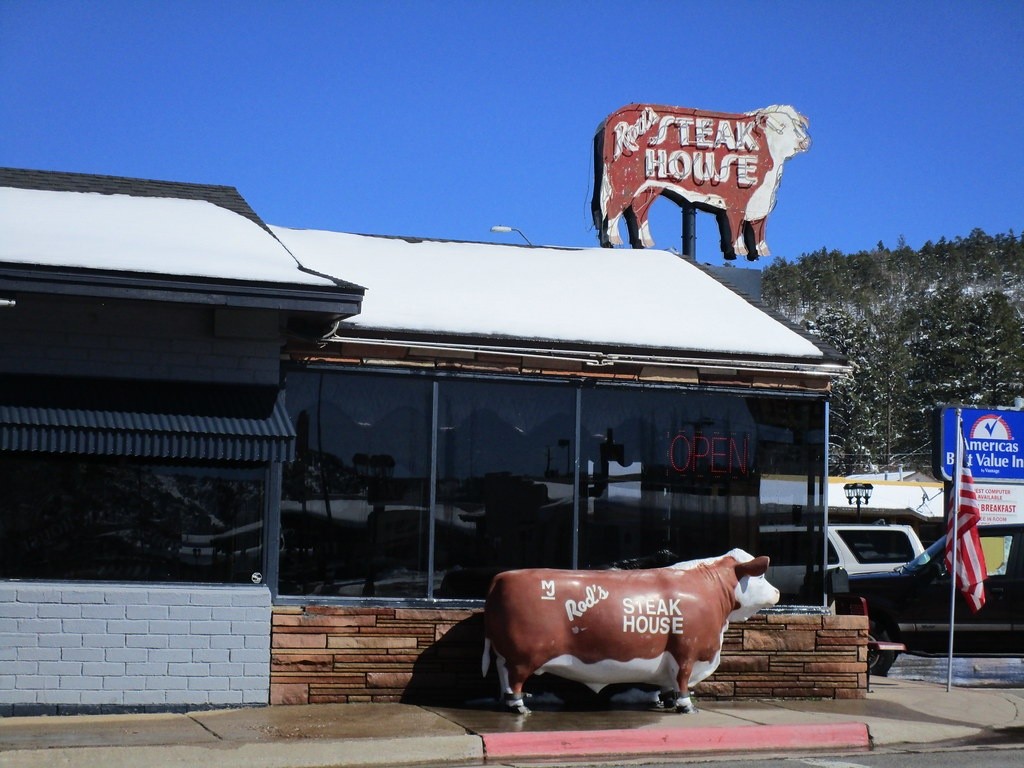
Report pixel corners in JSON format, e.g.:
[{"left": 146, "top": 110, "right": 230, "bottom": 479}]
[{"left": 944, "top": 413, "right": 986, "bottom": 612}]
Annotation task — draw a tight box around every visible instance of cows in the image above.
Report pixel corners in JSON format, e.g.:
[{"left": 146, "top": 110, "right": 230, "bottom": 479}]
[{"left": 481, "top": 548, "right": 781, "bottom": 715}]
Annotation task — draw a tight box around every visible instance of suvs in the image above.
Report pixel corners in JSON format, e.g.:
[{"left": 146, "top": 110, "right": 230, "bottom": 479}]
[
  {"left": 758, "top": 518, "right": 931, "bottom": 607},
  {"left": 801, "top": 522, "right": 1024, "bottom": 678}
]
